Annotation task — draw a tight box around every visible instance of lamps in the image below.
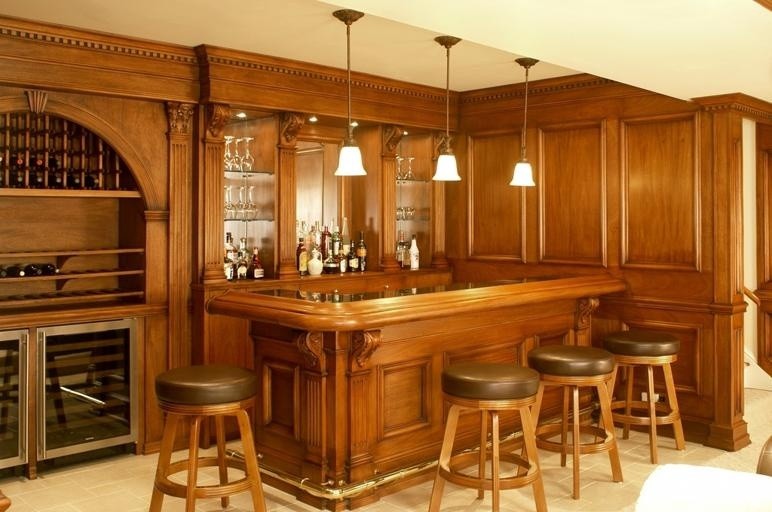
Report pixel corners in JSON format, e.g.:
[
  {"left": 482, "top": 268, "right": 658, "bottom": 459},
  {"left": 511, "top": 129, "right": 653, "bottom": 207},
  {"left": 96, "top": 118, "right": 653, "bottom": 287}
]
[
  {"left": 331, "top": 8, "right": 367, "bottom": 178},
  {"left": 507, "top": 57, "right": 540, "bottom": 188},
  {"left": 429, "top": 36, "right": 463, "bottom": 182}
]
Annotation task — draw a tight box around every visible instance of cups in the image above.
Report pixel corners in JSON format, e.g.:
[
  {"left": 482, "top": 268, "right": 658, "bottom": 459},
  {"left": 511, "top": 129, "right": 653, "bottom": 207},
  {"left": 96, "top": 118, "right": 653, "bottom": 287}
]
[{"left": 397, "top": 207, "right": 416, "bottom": 220}]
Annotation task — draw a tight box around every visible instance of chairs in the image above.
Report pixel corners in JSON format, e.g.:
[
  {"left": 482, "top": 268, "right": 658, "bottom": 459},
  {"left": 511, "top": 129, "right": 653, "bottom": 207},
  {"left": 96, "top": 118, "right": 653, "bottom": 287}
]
[{"left": 428, "top": 362, "right": 548, "bottom": 512}]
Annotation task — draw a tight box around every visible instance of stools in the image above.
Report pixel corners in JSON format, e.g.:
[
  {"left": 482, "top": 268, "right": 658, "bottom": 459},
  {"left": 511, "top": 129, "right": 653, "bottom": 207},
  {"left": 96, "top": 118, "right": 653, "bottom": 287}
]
[
  {"left": 149, "top": 362, "right": 267, "bottom": 511},
  {"left": 516, "top": 343, "right": 625, "bottom": 500},
  {"left": 593, "top": 328, "right": 686, "bottom": 466}
]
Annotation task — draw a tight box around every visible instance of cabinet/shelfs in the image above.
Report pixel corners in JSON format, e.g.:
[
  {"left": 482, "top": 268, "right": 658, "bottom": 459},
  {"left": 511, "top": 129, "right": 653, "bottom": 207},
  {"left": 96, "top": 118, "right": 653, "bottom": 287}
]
[
  {"left": 34, "top": 316, "right": 141, "bottom": 464},
  {"left": 1, "top": 324, "right": 31, "bottom": 472},
  {"left": 0, "top": 244, "right": 146, "bottom": 312},
  {"left": 223, "top": 166, "right": 275, "bottom": 225},
  {"left": 395, "top": 177, "right": 434, "bottom": 221}
]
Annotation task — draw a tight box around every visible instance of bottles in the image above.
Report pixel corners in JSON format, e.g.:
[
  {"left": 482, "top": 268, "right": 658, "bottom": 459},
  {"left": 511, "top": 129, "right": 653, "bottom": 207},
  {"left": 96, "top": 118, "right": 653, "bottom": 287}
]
[
  {"left": 341, "top": 217, "right": 351, "bottom": 256},
  {"left": 0, "top": 264, "right": 61, "bottom": 277},
  {"left": 296, "top": 216, "right": 348, "bottom": 276},
  {"left": 0, "top": 156, "right": 100, "bottom": 187},
  {"left": 224, "top": 232, "right": 265, "bottom": 281},
  {"left": 355, "top": 232, "right": 368, "bottom": 272},
  {"left": 396, "top": 232, "right": 419, "bottom": 269},
  {"left": 348, "top": 240, "right": 359, "bottom": 272}
]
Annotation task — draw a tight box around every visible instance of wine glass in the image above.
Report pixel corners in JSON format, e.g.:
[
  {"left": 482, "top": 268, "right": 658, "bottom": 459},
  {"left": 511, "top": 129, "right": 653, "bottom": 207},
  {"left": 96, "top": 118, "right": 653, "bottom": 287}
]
[
  {"left": 224, "top": 136, "right": 255, "bottom": 173},
  {"left": 223, "top": 185, "right": 260, "bottom": 218},
  {"left": 396, "top": 155, "right": 416, "bottom": 181}
]
